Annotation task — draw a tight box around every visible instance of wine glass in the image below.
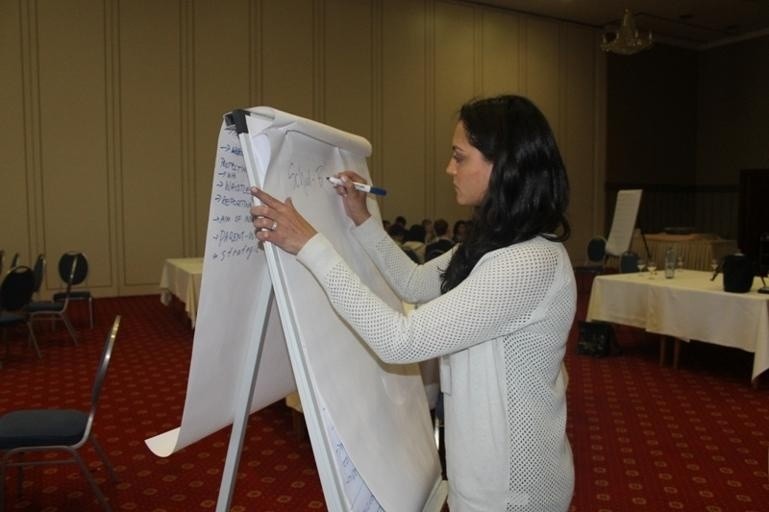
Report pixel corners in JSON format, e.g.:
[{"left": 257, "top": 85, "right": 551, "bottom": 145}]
[
  {"left": 637, "top": 258, "right": 645, "bottom": 277},
  {"left": 675, "top": 255, "right": 685, "bottom": 272},
  {"left": 647, "top": 259, "right": 658, "bottom": 281}
]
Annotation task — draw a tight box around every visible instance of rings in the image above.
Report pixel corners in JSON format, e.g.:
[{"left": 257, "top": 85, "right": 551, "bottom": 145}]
[{"left": 271, "top": 222, "right": 278, "bottom": 231}]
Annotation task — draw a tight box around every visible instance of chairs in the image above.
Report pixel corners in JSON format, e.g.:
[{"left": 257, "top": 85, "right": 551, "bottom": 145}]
[
  {"left": 619, "top": 250, "right": 643, "bottom": 274},
  {"left": 1, "top": 244, "right": 102, "bottom": 359},
  {"left": 3, "top": 314, "right": 124, "bottom": 509},
  {"left": 579, "top": 235, "right": 608, "bottom": 278}
]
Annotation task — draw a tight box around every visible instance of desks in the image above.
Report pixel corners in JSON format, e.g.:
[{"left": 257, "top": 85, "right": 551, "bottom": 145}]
[
  {"left": 586, "top": 266, "right": 768, "bottom": 392},
  {"left": 619, "top": 229, "right": 740, "bottom": 271},
  {"left": 160, "top": 256, "right": 206, "bottom": 329}
]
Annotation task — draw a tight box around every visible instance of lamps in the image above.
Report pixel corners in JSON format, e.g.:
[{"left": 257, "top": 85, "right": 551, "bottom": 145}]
[{"left": 598, "top": 2, "right": 659, "bottom": 61}]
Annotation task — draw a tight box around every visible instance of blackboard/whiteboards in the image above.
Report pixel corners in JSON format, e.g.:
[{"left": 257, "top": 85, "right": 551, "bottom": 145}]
[
  {"left": 231, "top": 105, "right": 443, "bottom": 512},
  {"left": 599, "top": 187, "right": 645, "bottom": 259}
]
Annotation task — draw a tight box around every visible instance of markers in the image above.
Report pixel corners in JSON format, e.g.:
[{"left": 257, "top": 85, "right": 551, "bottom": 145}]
[{"left": 325, "top": 176, "right": 388, "bottom": 198}]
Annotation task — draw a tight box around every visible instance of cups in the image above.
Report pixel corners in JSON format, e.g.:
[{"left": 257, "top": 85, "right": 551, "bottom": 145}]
[
  {"left": 710, "top": 257, "right": 720, "bottom": 270},
  {"left": 664, "top": 246, "right": 677, "bottom": 280}
]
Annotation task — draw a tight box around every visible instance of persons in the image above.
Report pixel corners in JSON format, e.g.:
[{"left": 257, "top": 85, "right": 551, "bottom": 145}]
[
  {"left": 250, "top": 95, "right": 579, "bottom": 512},
  {"left": 383, "top": 216, "right": 473, "bottom": 265}
]
